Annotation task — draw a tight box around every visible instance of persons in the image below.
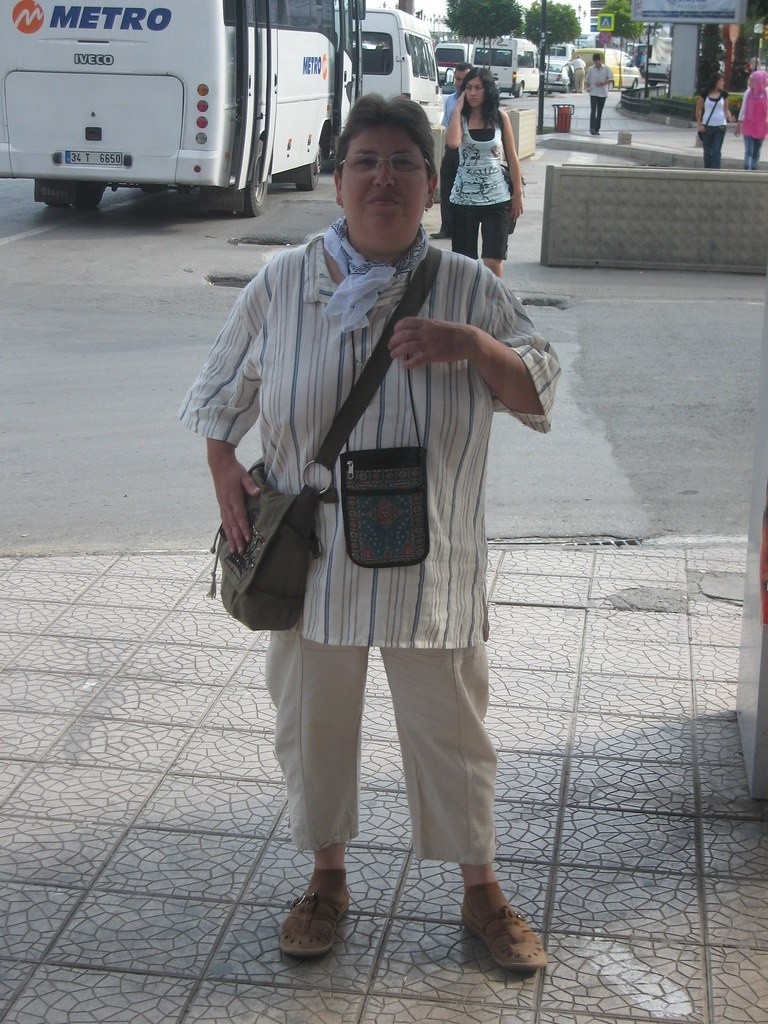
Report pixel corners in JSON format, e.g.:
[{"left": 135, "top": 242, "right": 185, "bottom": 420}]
[
  {"left": 695, "top": 73, "right": 736, "bottom": 168},
  {"left": 585, "top": 54, "right": 615, "bottom": 135},
  {"left": 428, "top": 63, "right": 473, "bottom": 238},
  {"left": 562, "top": 55, "right": 587, "bottom": 92},
  {"left": 178, "top": 94, "right": 560, "bottom": 969},
  {"left": 732, "top": 70, "right": 768, "bottom": 170},
  {"left": 445, "top": 67, "right": 525, "bottom": 278}
]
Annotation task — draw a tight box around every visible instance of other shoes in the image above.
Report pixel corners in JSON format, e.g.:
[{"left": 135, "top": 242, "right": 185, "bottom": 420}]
[
  {"left": 278, "top": 880, "right": 350, "bottom": 958},
  {"left": 460, "top": 892, "right": 547, "bottom": 971},
  {"left": 430, "top": 232, "right": 452, "bottom": 239}
]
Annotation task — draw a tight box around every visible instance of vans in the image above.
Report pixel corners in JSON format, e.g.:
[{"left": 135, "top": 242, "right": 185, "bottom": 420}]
[
  {"left": 349, "top": 7, "right": 455, "bottom": 128},
  {"left": 469, "top": 34, "right": 541, "bottom": 99},
  {"left": 431, "top": 41, "right": 474, "bottom": 94},
  {"left": 572, "top": 47, "right": 642, "bottom": 90},
  {"left": 535, "top": 41, "right": 575, "bottom": 62}
]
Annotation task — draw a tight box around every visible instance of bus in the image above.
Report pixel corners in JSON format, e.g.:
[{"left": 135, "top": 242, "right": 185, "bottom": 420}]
[{"left": 0, "top": 0, "right": 367, "bottom": 219}]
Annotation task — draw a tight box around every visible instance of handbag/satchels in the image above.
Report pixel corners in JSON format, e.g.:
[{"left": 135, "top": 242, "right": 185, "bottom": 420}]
[
  {"left": 698, "top": 129, "right": 704, "bottom": 142},
  {"left": 340, "top": 449, "right": 429, "bottom": 569},
  {"left": 207, "top": 455, "right": 318, "bottom": 632},
  {"left": 501, "top": 166, "right": 526, "bottom": 234}
]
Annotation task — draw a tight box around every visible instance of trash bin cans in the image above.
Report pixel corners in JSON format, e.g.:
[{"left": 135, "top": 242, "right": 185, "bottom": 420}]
[{"left": 553, "top": 104, "right": 575, "bottom": 133}]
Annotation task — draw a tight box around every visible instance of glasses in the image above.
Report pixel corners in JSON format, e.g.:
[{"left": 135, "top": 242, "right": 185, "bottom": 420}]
[{"left": 338, "top": 152, "right": 431, "bottom": 175}]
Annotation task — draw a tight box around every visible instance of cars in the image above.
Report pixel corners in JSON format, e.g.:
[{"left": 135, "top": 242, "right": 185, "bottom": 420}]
[{"left": 544, "top": 61, "right": 573, "bottom": 92}]
